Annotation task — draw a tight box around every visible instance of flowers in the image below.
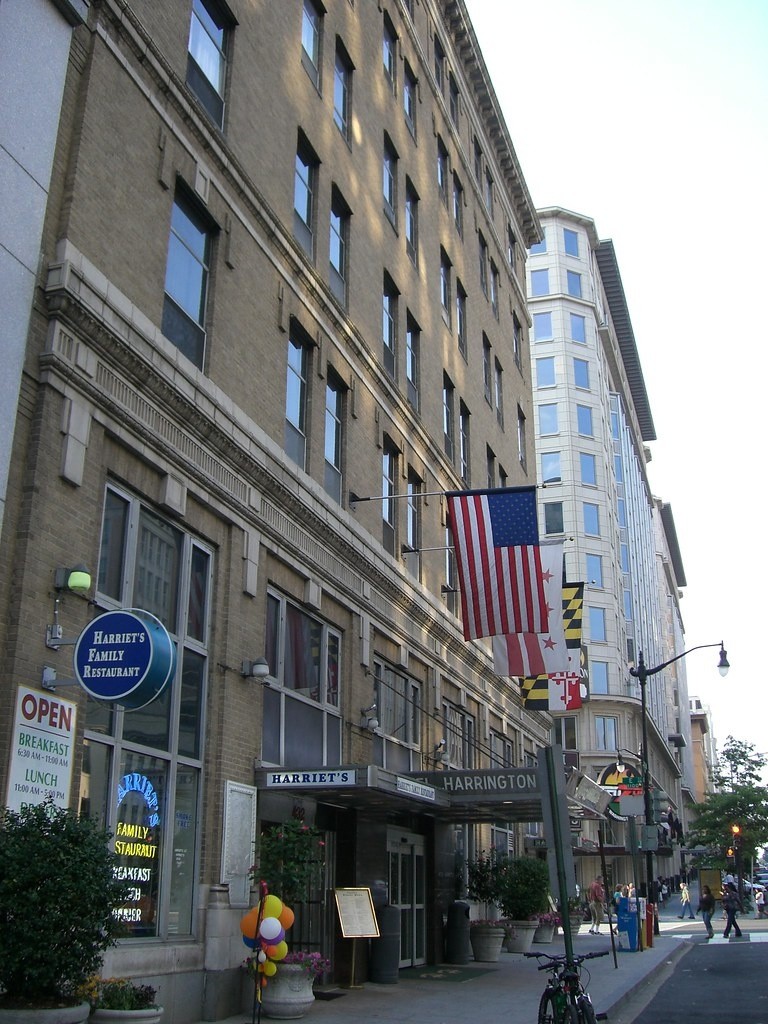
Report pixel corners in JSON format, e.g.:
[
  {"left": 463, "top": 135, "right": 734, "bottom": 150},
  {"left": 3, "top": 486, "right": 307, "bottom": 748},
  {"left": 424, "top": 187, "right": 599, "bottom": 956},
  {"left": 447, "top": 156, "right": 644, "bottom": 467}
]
[
  {"left": 77, "top": 975, "right": 165, "bottom": 1009},
  {"left": 534, "top": 912, "right": 562, "bottom": 925},
  {"left": 555, "top": 897, "right": 587, "bottom": 921},
  {"left": 242, "top": 814, "right": 332, "bottom": 982},
  {"left": 466, "top": 843, "right": 516, "bottom": 941}
]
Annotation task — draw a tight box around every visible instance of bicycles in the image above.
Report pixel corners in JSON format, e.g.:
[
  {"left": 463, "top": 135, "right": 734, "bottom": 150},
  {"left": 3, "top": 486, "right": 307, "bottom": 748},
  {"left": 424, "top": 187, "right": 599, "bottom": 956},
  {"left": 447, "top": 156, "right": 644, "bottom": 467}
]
[{"left": 523, "top": 951, "right": 609, "bottom": 1024}]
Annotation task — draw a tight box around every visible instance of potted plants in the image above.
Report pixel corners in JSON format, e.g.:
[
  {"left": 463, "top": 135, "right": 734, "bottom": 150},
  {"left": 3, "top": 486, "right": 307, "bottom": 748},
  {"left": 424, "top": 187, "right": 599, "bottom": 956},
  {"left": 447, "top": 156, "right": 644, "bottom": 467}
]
[
  {"left": 0, "top": 798, "right": 128, "bottom": 1024},
  {"left": 494, "top": 855, "right": 550, "bottom": 953}
]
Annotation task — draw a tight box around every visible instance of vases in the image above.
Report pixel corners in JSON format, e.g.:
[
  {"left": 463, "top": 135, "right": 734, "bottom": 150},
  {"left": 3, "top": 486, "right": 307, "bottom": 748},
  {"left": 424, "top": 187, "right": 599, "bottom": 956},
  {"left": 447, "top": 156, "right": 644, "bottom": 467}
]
[
  {"left": 468, "top": 928, "right": 507, "bottom": 962},
  {"left": 90, "top": 1004, "right": 164, "bottom": 1023},
  {"left": 261, "top": 961, "right": 320, "bottom": 1018},
  {"left": 560, "top": 915, "right": 581, "bottom": 937},
  {"left": 534, "top": 923, "right": 556, "bottom": 943}
]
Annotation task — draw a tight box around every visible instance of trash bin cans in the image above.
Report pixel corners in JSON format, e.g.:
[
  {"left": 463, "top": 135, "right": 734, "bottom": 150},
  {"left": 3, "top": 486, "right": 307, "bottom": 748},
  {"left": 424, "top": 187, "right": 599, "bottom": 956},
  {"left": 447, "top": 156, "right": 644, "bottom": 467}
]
[
  {"left": 448, "top": 899, "right": 474, "bottom": 964},
  {"left": 370, "top": 904, "right": 401, "bottom": 983}
]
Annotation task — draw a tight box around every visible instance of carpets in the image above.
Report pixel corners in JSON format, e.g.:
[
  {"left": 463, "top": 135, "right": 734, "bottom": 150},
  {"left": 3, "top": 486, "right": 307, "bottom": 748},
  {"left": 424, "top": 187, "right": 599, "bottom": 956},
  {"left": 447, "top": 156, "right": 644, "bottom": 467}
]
[{"left": 314, "top": 990, "right": 346, "bottom": 1002}]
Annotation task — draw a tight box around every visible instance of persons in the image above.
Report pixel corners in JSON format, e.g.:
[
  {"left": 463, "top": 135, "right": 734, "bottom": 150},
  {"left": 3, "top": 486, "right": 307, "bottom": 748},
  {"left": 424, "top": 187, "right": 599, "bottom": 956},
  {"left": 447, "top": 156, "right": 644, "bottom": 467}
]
[
  {"left": 589, "top": 875, "right": 605, "bottom": 936},
  {"left": 609, "top": 876, "right": 670, "bottom": 935},
  {"left": 754, "top": 888, "right": 768, "bottom": 919},
  {"left": 696, "top": 885, "right": 715, "bottom": 939},
  {"left": 719, "top": 882, "right": 746, "bottom": 938},
  {"left": 677, "top": 883, "right": 695, "bottom": 919}
]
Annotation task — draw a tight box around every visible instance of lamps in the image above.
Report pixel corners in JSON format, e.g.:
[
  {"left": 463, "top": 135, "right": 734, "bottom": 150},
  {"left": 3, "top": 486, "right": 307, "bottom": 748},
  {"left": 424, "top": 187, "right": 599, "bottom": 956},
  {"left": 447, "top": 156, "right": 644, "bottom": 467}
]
[
  {"left": 240, "top": 656, "right": 270, "bottom": 680},
  {"left": 52, "top": 564, "right": 93, "bottom": 592},
  {"left": 434, "top": 739, "right": 446, "bottom": 750},
  {"left": 360, "top": 704, "right": 379, "bottom": 728},
  {"left": 435, "top": 750, "right": 450, "bottom": 761}
]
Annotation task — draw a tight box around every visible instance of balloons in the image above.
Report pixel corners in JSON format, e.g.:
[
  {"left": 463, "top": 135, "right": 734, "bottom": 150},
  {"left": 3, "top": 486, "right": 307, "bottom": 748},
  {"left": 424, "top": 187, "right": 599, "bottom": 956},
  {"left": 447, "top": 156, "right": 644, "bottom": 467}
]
[{"left": 240, "top": 894, "right": 295, "bottom": 988}]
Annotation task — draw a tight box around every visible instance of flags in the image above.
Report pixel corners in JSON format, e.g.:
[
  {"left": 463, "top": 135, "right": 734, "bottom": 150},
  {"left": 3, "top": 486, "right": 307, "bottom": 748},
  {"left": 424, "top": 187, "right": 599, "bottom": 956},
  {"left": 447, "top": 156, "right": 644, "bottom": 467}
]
[
  {"left": 518, "top": 580, "right": 585, "bottom": 711},
  {"left": 492, "top": 538, "right": 571, "bottom": 677},
  {"left": 444, "top": 485, "right": 550, "bottom": 642}
]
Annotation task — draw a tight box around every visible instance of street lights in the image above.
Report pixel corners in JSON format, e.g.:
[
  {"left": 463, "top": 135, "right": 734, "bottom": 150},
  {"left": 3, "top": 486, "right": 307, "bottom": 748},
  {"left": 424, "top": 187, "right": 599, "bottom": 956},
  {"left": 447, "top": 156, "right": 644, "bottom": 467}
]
[{"left": 616, "top": 641, "right": 730, "bottom": 935}]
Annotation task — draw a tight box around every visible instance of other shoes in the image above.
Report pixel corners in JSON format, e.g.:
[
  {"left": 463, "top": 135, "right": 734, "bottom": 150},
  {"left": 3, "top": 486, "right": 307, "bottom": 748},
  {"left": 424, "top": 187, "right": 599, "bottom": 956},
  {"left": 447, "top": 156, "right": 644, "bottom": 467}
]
[
  {"left": 705, "top": 933, "right": 714, "bottom": 939},
  {"left": 677, "top": 915, "right": 683, "bottom": 919},
  {"left": 688, "top": 916, "right": 695, "bottom": 919},
  {"left": 595, "top": 932, "right": 604, "bottom": 936},
  {"left": 589, "top": 930, "right": 596, "bottom": 935}
]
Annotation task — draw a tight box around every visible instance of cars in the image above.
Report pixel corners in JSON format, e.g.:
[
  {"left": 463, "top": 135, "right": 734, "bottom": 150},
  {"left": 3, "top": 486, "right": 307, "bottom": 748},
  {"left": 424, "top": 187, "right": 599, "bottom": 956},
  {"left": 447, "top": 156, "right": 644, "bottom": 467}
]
[
  {"left": 734, "top": 877, "right": 765, "bottom": 894},
  {"left": 756, "top": 874, "right": 768, "bottom": 888},
  {"left": 746, "top": 867, "right": 768, "bottom": 883}
]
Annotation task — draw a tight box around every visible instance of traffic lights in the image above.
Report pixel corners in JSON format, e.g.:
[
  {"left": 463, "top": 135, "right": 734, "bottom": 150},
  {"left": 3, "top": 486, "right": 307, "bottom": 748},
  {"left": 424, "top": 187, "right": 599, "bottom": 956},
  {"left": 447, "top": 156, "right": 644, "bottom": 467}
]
[
  {"left": 733, "top": 827, "right": 740, "bottom": 846},
  {"left": 654, "top": 789, "right": 668, "bottom": 822}
]
